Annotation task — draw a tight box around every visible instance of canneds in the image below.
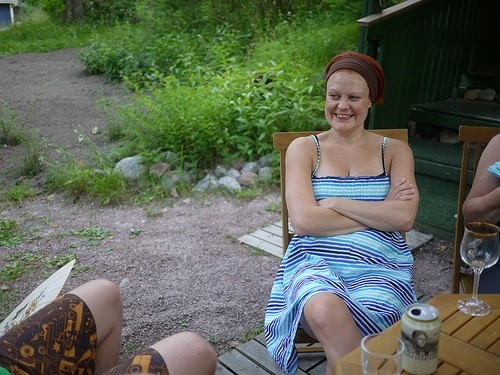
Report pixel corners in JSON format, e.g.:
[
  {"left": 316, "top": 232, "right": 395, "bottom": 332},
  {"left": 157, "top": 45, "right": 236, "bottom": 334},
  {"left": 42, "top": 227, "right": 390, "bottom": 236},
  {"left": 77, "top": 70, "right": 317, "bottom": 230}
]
[{"left": 397, "top": 303, "right": 441, "bottom": 375}]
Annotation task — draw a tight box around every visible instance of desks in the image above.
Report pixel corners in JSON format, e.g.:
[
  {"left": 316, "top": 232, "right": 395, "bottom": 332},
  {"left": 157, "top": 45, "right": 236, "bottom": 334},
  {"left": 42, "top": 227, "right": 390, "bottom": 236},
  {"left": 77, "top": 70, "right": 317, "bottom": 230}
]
[
  {"left": 409, "top": 100, "right": 500, "bottom": 137},
  {"left": 336, "top": 294, "right": 500, "bottom": 375}
]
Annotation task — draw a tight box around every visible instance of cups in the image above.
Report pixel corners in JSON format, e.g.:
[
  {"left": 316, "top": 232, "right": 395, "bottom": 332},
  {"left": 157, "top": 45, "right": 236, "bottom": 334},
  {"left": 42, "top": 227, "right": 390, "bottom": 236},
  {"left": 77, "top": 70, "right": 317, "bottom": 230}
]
[{"left": 361, "top": 333, "right": 405, "bottom": 375}]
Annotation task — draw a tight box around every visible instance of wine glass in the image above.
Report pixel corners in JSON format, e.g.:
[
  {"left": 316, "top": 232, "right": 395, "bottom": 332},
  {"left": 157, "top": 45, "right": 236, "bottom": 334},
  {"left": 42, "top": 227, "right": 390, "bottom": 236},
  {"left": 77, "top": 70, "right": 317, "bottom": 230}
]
[{"left": 456, "top": 222, "right": 500, "bottom": 316}]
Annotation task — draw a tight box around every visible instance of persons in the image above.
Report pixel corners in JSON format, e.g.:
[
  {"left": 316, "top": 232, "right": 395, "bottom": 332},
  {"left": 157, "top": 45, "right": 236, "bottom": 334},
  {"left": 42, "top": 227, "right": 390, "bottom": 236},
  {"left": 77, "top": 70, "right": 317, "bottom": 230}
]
[
  {"left": 264, "top": 51, "right": 419, "bottom": 375},
  {"left": 462, "top": 133, "right": 500, "bottom": 294},
  {"left": 0, "top": 277, "right": 217, "bottom": 375}
]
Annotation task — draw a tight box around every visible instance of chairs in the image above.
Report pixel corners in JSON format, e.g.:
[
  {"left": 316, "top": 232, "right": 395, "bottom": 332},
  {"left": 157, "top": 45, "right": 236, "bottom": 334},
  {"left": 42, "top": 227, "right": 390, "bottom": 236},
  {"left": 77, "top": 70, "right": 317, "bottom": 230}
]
[
  {"left": 452, "top": 125, "right": 500, "bottom": 294},
  {"left": 272, "top": 129, "right": 408, "bottom": 354}
]
